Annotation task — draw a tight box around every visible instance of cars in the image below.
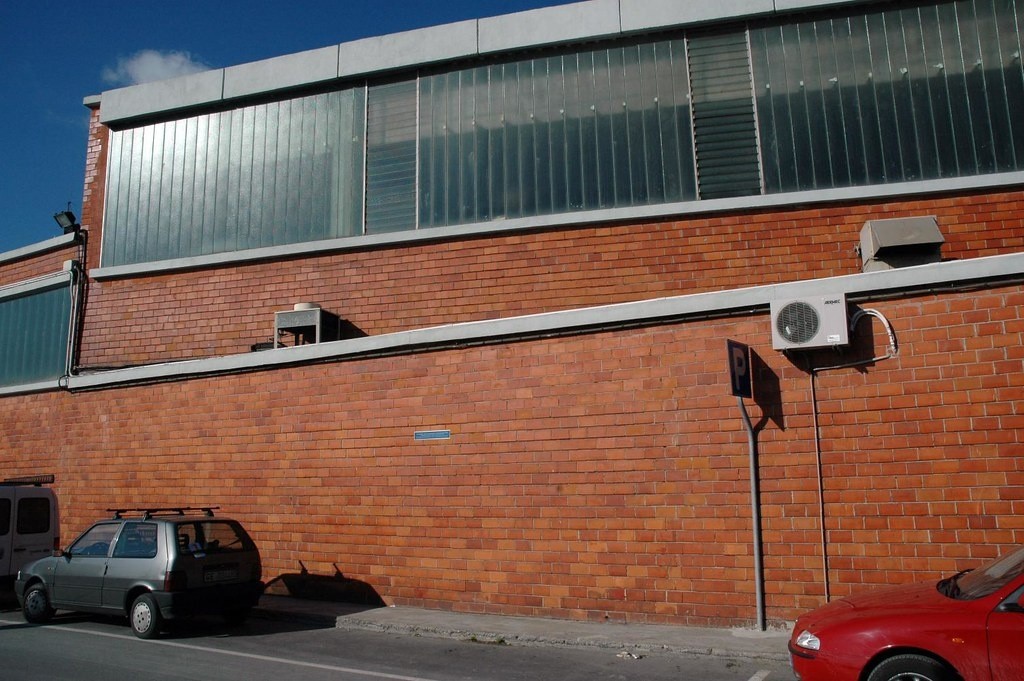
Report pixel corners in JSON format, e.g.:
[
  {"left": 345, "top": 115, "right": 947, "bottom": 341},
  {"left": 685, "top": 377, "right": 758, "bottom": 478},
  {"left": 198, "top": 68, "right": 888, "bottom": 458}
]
[{"left": 789, "top": 544, "right": 1024, "bottom": 681}]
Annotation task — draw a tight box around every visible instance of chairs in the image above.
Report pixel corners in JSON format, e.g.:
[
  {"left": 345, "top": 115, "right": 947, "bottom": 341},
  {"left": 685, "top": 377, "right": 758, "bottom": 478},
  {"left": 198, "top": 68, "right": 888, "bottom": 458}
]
[{"left": 122, "top": 532, "right": 142, "bottom": 552}]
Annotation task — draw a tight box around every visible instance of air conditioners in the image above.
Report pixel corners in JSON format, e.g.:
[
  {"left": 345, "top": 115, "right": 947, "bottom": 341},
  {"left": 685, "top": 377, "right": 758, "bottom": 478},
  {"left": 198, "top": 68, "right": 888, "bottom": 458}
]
[{"left": 769, "top": 292, "right": 849, "bottom": 351}]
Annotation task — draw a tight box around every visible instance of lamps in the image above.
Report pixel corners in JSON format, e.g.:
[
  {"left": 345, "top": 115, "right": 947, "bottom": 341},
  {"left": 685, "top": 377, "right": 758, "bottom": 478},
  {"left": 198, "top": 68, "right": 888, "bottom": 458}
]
[
  {"left": 870, "top": 217, "right": 945, "bottom": 257},
  {"left": 53, "top": 212, "right": 80, "bottom": 234}
]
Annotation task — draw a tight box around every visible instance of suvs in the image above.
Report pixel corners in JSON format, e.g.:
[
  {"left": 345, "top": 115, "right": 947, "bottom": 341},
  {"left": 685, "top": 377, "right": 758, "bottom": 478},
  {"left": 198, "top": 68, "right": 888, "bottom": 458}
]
[{"left": 16, "top": 507, "right": 264, "bottom": 639}]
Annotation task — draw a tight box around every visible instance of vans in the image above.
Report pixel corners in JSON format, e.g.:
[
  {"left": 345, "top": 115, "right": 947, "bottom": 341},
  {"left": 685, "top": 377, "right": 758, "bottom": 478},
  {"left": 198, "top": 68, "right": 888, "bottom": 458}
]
[{"left": 0, "top": 473, "right": 60, "bottom": 608}]
